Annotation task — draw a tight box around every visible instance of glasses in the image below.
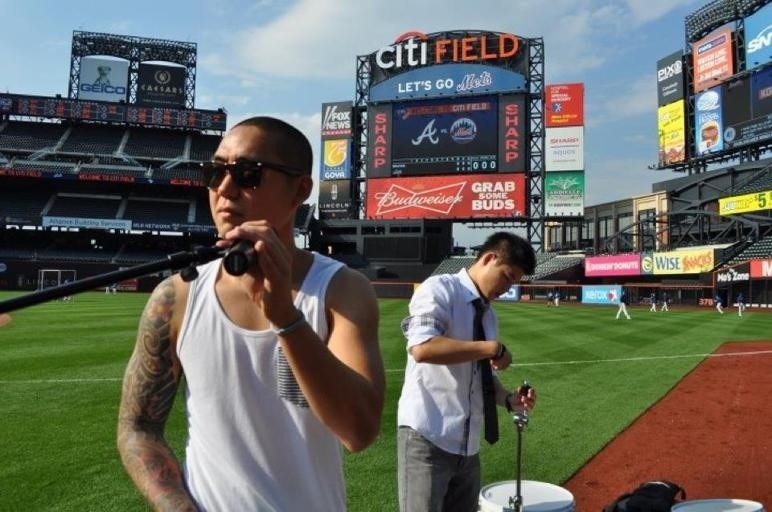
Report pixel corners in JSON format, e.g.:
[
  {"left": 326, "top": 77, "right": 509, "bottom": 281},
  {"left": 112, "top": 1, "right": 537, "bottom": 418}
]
[{"left": 200, "top": 159, "right": 300, "bottom": 188}]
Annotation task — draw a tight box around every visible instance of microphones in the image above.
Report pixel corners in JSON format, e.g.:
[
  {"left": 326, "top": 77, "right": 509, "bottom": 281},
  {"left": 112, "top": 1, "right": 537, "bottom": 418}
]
[{"left": 221, "top": 224, "right": 279, "bottom": 279}]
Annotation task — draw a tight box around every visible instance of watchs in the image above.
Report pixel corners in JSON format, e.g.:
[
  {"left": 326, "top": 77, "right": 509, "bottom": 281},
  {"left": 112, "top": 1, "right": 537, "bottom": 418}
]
[{"left": 492, "top": 343, "right": 507, "bottom": 361}]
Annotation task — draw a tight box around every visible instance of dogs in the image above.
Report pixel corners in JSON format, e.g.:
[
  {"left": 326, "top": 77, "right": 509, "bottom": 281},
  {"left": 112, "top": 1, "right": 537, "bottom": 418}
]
[{"left": 95, "top": 65, "right": 112, "bottom": 86}]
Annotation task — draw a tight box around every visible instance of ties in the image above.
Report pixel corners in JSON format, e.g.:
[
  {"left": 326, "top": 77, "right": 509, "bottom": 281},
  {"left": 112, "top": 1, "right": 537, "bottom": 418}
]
[{"left": 473, "top": 298, "right": 499, "bottom": 444}]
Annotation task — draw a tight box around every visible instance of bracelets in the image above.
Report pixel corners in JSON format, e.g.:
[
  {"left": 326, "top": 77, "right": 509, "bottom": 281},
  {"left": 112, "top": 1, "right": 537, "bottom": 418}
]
[
  {"left": 269, "top": 311, "right": 306, "bottom": 337},
  {"left": 504, "top": 393, "right": 514, "bottom": 414}
]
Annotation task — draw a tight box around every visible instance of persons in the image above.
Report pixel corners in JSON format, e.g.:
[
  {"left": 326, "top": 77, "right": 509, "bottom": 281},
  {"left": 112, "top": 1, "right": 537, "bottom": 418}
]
[
  {"left": 615, "top": 290, "right": 631, "bottom": 320},
  {"left": 105, "top": 286, "right": 110, "bottom": 294},
  {"left": 115, "top": 115, "right": 389, "bottom": 512},
  {"left": 553, "top": 290, "right": 560, "bottom": 307},
  {"left": 661, "top": 292, "right": 669, "bottom": 312},
  {"left": 735, "top": 288, "right": 746, "bottom": 317},
  {"left": 713, "top": 295, "right": 724, "bottom": 315},
  {"left": 546, "top": 291, "right": 553, "bottom": 305},
  {"left": 395, "top": 230, "right": 538, "bottom": 512},
  {"left": 650, "top": 293, "right": 657, "bottom": 312},
  {"left": 111, "top": 283, "right": 117, "bottom": 295}
]
